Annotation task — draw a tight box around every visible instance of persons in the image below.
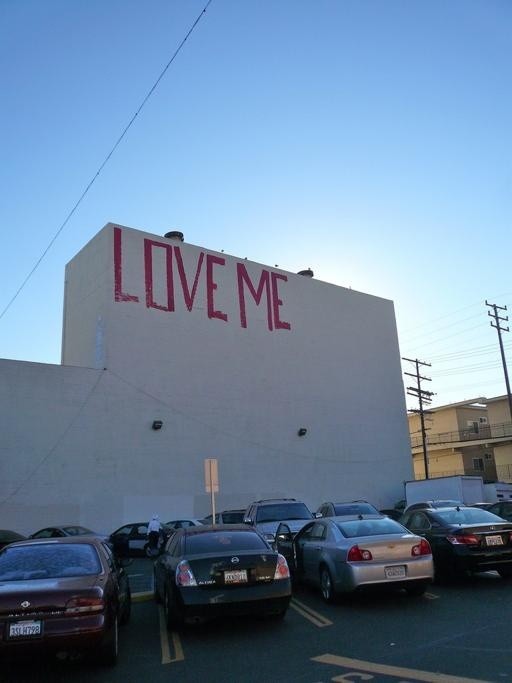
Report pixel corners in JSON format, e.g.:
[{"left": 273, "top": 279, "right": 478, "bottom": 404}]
[{"left": 146, "top": 513, "right": 162, "bottom": 557}]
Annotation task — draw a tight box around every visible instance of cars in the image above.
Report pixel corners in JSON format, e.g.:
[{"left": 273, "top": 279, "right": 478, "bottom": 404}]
[
  {"left": 0, "top": 525, "right": 132, "bottom": 668},
  {"left": 154, "top": 523, "right": 293, "bottom": 636},
  {"left": 243, "top": 498, "right": 512, "bottom": 601},
  {"left": 108, "top": 509, "right": 246, "bottom": 557}
]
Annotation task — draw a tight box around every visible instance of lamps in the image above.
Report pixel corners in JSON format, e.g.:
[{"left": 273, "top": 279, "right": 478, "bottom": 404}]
[
  {"left": 298, "top": 428, "right": 307, "bottom": 437},
  {"left": 152, "top": 420, "right": 163, "bottom": 429}
]
